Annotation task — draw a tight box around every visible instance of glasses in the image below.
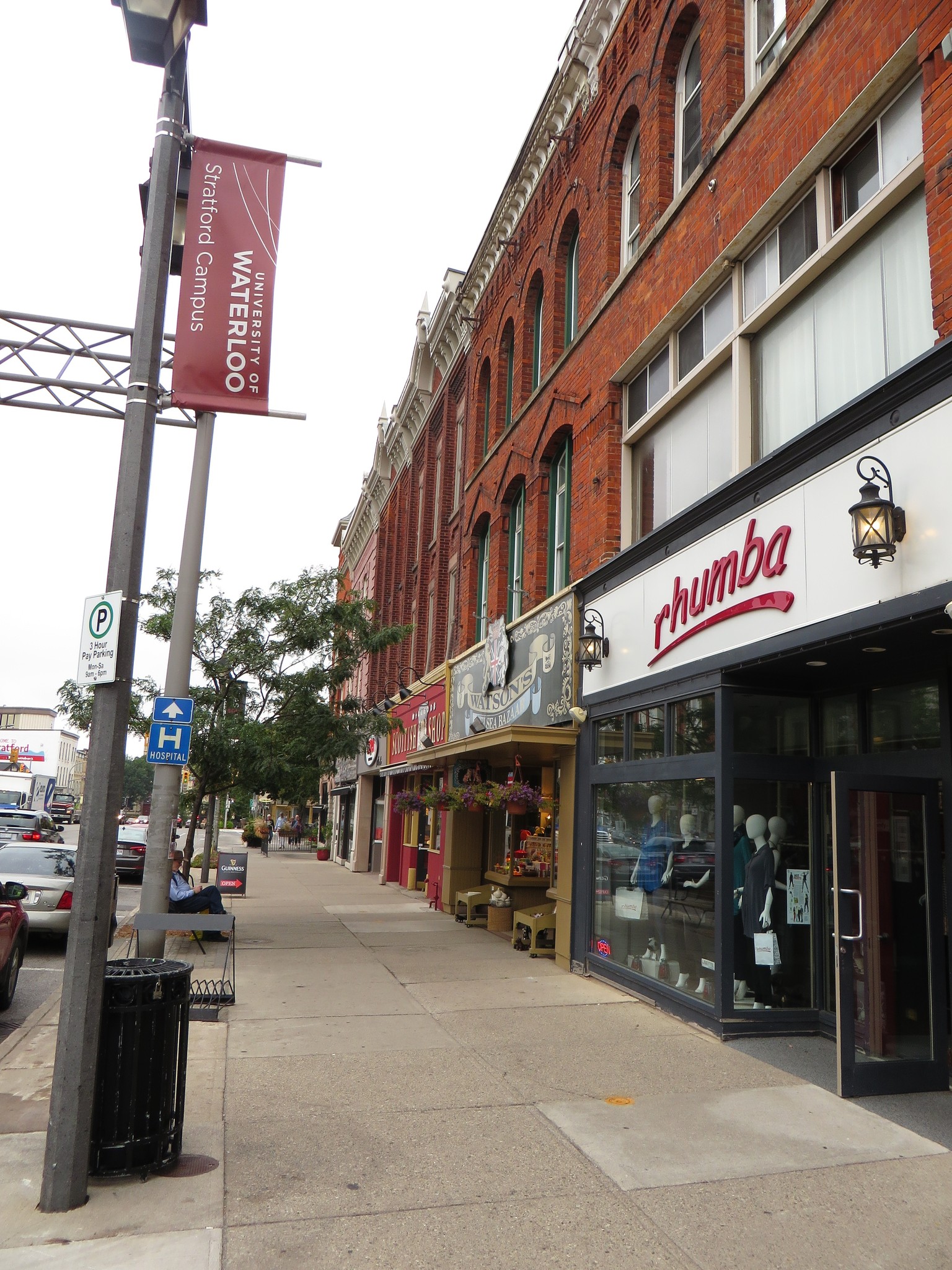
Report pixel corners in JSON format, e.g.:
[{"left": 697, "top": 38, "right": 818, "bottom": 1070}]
[{"left": 175, "top": 859, "right": 181, "bottom": 864}]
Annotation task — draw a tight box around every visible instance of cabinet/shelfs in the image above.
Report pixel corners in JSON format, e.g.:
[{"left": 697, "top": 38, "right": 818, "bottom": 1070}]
[
  {"left": 511, "top": 901, "right": 557, "bottom": 958},
  {"left": 454, "top": 884, "right": 504, "bottom": 928}
]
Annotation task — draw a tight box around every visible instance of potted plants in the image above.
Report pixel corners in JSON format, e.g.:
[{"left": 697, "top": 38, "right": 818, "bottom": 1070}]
[{"left": 317, "top": 847, "right": 331, "bottom": 860}]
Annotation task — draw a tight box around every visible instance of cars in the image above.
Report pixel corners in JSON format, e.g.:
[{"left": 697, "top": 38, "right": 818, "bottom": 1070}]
[
  {"left": 0, "top": 882, "right": 30, "bottom": 1012},
  {"left": 115, "top": 825, "right": 150, "bottom": 877},
  {"left": 118, "top": 813, "right": 126, "bottom": 825},
  {"left": 135, "top": 815, "right": 149, "bottom": 824},
  {"left": 126, "top": 818, "right": 136, "bottom": 824},
  {"left": 598, "top": 843, "right": 657, "bottom": 894},
  {"left": 0, "top": 841, "right": 121, "bottom": 947},
  {"left": 177, "top": 815, "right": 183, "bottom": 828},
  {"left": 185, "top": 819, "right": 198, "bottom": 829}
]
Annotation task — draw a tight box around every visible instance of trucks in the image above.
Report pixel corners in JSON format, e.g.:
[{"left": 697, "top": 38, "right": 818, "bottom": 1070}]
[{"left": 0, "top": 770, "right": 56, "bottom": 815}]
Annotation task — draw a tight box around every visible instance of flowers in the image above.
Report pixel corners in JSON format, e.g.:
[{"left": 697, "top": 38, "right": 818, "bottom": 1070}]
[
  {"left": 384, "top": 790, "right": 422, "bottom": 814},
  {"left": 448, "top": 783, "right": 495, "bottom": 808},
  {"left": 416, "top": 784, "right": 453, "bottom": 806},
  {"left": 487, "top": 779, "right": 542, "bottom": 808}
]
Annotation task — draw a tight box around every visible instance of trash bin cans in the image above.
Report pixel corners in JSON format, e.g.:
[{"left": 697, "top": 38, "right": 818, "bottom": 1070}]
[{"left": 86, "top": 956, "right": 195, "bottom": 1183}]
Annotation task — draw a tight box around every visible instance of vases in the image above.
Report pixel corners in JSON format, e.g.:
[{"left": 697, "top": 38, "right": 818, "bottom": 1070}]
[
  {"left": 503, "top": 801, "right": 523, "bottom": 813},
  {"left": 406, "top": 804, "right": 417, "bottom": 810},
  {"left": 466, "top": 800, "right": 479, "bottom": 812},
  {"left": 435, "top": 803, "right": 450, "bottom": 811}
]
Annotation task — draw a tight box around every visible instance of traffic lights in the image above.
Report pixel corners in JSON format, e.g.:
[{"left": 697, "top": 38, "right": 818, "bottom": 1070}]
[
  {"left": 230, "top": 799, "right": 235, "bottom": 804},
  {"left": 182, "top": 771, "right": 189, "bottom": 784}
]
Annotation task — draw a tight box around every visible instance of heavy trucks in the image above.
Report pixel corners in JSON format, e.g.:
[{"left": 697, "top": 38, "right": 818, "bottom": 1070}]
[{"left": 51, "top": 792, "right": 81, "bottom": 824}]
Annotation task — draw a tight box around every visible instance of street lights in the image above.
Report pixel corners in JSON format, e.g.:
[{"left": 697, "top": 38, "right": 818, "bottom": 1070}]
[{"left": 108, "top": 0, "right": 223, "bottom": 958}]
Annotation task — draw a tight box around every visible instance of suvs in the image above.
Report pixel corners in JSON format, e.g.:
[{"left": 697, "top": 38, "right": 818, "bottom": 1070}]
[
  {"left": 597, "top": 825, "right": 615, "bottom": 845},
  {"left": 0, "top": 807, "right": 65, "bottom": 849},
  {"left": 610, "top": 829, "right": 647, "bottom": 848},
  {"left": 199, "top": 818, "right": 207, "bottom": 829},
  {"left": 641, "top": 835, "right": 715, "bottom": 889}
]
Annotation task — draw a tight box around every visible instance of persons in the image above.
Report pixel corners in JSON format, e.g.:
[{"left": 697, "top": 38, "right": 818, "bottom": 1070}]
[
  {"left": 291, "top": 815, "right": 300, "bottom": 846},
  {"left": 274, "top": 812, "right": 288, "bottom": 849},
  {"left": 288, "top": 817, "right": 294, "bottom": 846},
  {"left": 264, "top": 814, "right": 275, "bottom": 844},
  {"left": 168, "top": 850, "right": 230, "bottom": 942},
  {"left": 621, "top": 791, "right": 806, "bottom": 1014},
  {"left": 291, "top": 818, "right": 304, "bottom": 850}
]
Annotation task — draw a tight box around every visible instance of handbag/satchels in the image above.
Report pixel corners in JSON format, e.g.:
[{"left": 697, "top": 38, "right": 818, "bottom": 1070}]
[
  {"left": 753, "top": 921, "right": 782, "bottom": 966},
  {"left": 614, "top": 881, "right": 649, "bottom": 922}
]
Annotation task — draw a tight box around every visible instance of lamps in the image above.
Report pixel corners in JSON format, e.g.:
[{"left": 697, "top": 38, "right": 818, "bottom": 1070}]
[
  {"left": 398, "top": 666, "right": 446, "bottom": 702},
  {"left": 849, "top": 456, "right": 906, "bottom": 569},
  {"left": 383, "top": 681, "right": 426, "bottom": 713},
  {"left": 569, "top": 707, "right": 587, "bottom": 724},
  {"left": 371, "top": 692, "right": 410, "bottom": 716},
  {"left": 469, "top": 716, "right": 486, "bottom": 734},
  {"left": 578, "top": 609, "right": 609, "bottom": 672},
  {"left": 421, "top": 735, "right": 434, "bottom": 748},
  {"left": 361, "top": 699, "right": 396, "bottom": 722}
]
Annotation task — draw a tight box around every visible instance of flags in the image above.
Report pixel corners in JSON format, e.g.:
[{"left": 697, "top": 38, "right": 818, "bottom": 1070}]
[{"left": 230, "top": 798, "right": 235, "bottom": 804}]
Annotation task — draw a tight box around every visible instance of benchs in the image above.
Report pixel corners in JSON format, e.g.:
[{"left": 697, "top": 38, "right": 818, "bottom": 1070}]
[
  {"left": 171, "top": 875, "right": 210, "bottom": 956},
  {"left": 661, "top": 872, "right": 715, "bottom": 934}
]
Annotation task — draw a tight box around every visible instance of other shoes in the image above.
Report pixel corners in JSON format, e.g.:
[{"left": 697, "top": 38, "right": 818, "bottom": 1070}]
[
  {"left": 279, "top": 847, "right": 282, "bottom": 848},
  {"left": 269, "top": 840, "right": 271, "bottom": 843},
  {"left": 283, "top": 846, "right": 285, "bottom": 848},
  {"left": 202, "top": 932, "right": 229, "bottom": 942},
  {"left": 298, "top": 846, "right": 300, "bottom": 849},
  {"left": 295, "top": 846, "right": 297, "bottom": 849}
]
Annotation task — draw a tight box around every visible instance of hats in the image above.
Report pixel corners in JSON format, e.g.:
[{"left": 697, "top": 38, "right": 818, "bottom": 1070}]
[
  {"left": 280, "top": 812, "right": 283, "bottom": 815},
  {"left": 174, "top": 850, "right": 189, "bottom": 862}
]
